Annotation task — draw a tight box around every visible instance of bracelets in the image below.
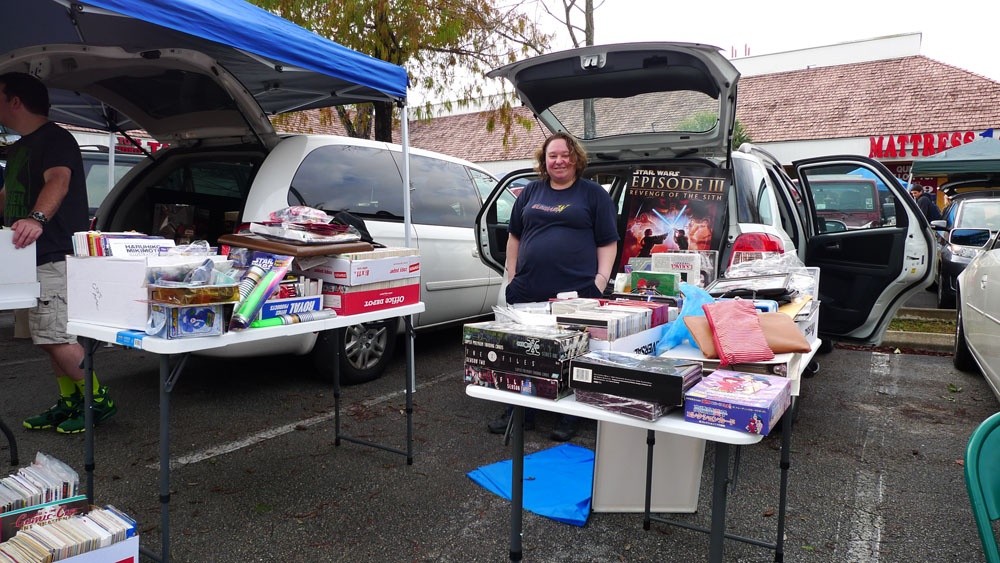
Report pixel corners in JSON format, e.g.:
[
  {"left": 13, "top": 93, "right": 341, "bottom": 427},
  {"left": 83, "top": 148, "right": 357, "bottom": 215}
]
[{"left": 596, "top": 273, "right": 607, "bottom": 284}]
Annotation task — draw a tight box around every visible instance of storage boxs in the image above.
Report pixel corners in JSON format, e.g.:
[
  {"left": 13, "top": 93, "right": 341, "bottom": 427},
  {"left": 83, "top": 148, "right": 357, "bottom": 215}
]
[
  {"left": 462, "top": 324, "right": 791, "bottom": 437},
  {"left": 65, "top": 255, "right": 154, "bottom": 331},
  {"left": 151, "top": 286, "right": 240, "bottom": 336},
  {"left": 293, "top": 257, "right": 422, "bottom": 315},
  {"left": 0, "top": 229, "right": 40, "bottom": 299},
  {"left": 50, "top": 504, "right": 139, "bottom": 563}
]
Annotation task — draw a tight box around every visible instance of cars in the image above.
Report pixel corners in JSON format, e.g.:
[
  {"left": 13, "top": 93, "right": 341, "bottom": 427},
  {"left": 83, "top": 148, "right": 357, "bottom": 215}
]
[{"left": 929, "top": 190, "right": 1000, "bottom": 404}]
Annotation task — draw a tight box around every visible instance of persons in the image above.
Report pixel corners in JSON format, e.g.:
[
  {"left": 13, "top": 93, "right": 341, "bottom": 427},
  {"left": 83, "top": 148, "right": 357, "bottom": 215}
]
[
  {"left": 486, "top": 132, "right": 621, "bottom": 441},
  {"left": 639, "top": 228, "right": 708, "bottom": 289},
  {"left": 0, "top": 73, "right": 118, "bottom": 435},
  {"left": 910, "top": 185, "right": 950, "bottom": 291}
]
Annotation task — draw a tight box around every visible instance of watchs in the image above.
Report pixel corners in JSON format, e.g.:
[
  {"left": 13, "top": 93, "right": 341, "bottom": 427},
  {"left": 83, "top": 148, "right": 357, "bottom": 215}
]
[{"left": 27, "top": 211, "right": 49, "bottom": 227}]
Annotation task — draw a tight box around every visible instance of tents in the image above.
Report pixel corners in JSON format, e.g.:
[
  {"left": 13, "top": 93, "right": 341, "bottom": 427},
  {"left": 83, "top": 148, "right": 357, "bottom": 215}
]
[
  {"left": 834, "top": 138, "right": 1000, "bottom": 206},
  {"left": 0, "top": 0, "right": 418, "bottom": 394}
]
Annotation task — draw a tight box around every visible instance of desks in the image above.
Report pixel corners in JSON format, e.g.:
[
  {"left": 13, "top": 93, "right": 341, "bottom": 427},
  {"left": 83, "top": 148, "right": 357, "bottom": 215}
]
[
  {"left": 474, "top": 336, "right": 821, "bottom": 562},
  {"left": 65, "top": 303, "right": 426, "bottom": 561}
]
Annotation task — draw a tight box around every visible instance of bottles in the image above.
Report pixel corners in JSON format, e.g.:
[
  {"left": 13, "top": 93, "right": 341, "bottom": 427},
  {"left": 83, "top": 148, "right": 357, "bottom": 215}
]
[{"left": 182, "top": 259, "right": 215, "bottom": 285}]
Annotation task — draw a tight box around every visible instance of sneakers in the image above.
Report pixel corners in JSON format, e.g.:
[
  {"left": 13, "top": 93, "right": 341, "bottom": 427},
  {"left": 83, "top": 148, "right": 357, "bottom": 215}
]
[
  {"left": 57, "top": 386, "right": 118, "bottom": 435},
  {"left": 22, "top": 385, "right": 88, "bottom": 430},
  {"left": 551, "top": 418, "right": 577, "bottom": 442},
  {"left": 488, "top": 413, "right": 537, "bottom": 433}
]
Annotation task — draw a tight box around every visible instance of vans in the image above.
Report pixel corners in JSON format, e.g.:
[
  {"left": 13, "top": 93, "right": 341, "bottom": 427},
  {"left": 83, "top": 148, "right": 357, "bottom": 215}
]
[{"left": 72, "top": 133, "right": 521, "bottom": 387}]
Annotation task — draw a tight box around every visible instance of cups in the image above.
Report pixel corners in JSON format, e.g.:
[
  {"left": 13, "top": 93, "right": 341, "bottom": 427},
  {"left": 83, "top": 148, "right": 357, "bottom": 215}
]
[{"left": 233, "top": 262, "right": 337, "bottom": 329}]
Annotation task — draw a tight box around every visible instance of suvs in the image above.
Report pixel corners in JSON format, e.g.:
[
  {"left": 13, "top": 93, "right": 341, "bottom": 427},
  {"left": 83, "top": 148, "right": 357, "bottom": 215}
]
[{"left": 475, "top": 42, "right": 938, "bottom": 352}]
